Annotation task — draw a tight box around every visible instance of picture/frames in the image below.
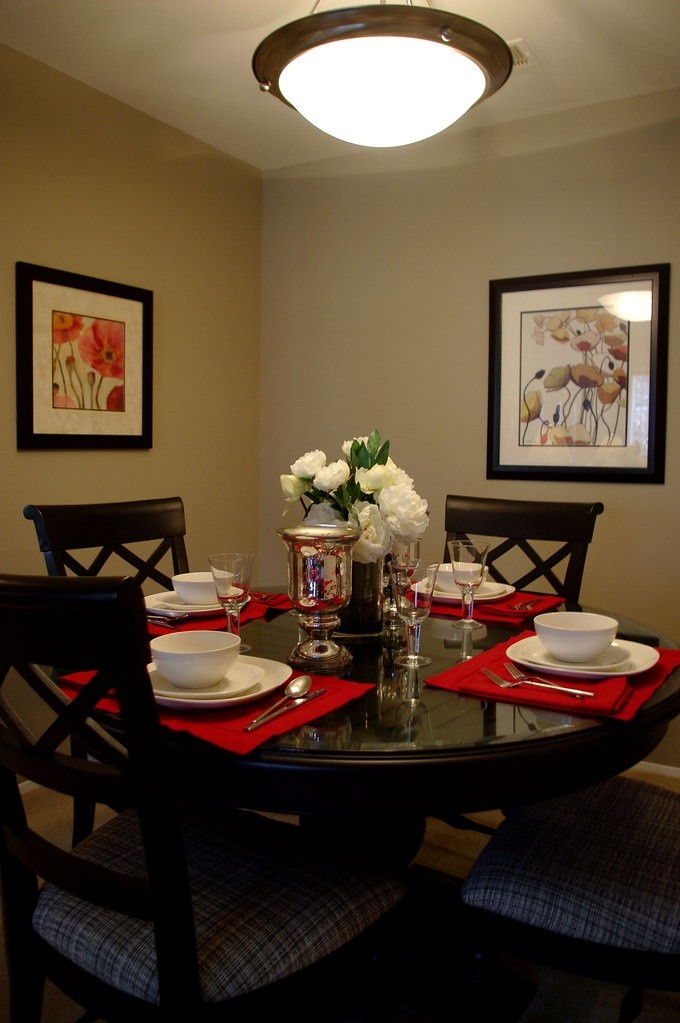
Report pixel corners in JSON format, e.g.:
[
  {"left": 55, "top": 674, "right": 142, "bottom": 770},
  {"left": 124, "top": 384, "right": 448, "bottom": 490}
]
[
  {"left": 14, "top": 260, "right": 155, "bottom": 450},
  {"left": 485, "top": 263, "right": 670, "bottom": 488}
]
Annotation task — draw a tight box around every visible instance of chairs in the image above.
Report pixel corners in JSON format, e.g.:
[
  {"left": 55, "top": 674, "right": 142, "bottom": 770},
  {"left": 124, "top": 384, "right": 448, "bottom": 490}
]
[
  {"left": 465, "top": 778, "right": 680, "bottom": 1023},
  {"left": 440, "top": 494, "right": 606, "bottom": 837},
  {"left": 24, "top": 498, "right": 190, "bottom": 847},
  {"left": 0, "top": 574, "right": 426, "bottom": 1023}
]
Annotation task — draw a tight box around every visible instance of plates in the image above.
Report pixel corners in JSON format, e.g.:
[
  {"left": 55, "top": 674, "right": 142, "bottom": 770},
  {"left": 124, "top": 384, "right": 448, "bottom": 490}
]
[
  {"left": 506, "top": 634, "right": 660, "bottom": 678},
  {"left": 141, "top": 586, "right": 251, "bottom": 616},
  {"left": 139, "top": 652, "right": 296, "bottom": 709},
  {"left": 411, "top": 579, "right": 516, "bottom": 605}
]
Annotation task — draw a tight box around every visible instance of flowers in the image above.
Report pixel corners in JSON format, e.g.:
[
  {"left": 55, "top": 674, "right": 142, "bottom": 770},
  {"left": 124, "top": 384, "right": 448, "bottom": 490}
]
[{"left": 279, "top": 428, "right": 429, "bottom": 560}]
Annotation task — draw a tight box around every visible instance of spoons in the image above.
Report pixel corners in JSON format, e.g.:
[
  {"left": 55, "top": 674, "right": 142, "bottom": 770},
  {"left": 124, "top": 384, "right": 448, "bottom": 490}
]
[
  {"left": 250, "top": 676, "right": 313, "bottom": 723},
  {"left": 146, "top": 614, "right": 191, "bottom": 623}
]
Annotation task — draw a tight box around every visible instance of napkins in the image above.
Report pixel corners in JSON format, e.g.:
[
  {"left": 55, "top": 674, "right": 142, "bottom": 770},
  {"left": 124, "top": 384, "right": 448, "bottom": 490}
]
[
  {"left": 477, "top": 594, "right": 566, "bottom": 618},
  {"left": 460, "top": 656, "right": 636, "bottom": 715}
]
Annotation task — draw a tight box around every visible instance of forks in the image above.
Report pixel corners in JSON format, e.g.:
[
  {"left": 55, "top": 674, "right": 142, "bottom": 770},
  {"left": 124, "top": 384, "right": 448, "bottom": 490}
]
[
  {"left": 503, "top": 661, "right": 584, "bottom": 699},
  {"left": 482, "top": 666, "right": 594, "bottom": 699}
]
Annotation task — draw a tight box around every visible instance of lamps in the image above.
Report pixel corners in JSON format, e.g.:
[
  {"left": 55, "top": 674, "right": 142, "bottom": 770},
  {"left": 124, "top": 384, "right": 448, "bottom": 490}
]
[{"left": 250, "top": 6, "right": 515, "bottom": 151}]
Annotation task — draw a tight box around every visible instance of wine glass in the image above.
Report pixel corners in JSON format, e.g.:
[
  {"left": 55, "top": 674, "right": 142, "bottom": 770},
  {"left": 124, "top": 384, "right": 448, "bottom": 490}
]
[
  {"left": 209, "top": 553, "right": 254, "bottom": 655},
  {"left": 386, "top": 555, "right": 433, "bottom": 666},
  {"left": 393, "top": 669, "right": 434, "bottom": 743},
  {"left": 447, "top": 540, "right": 490, "bottom": 629}
]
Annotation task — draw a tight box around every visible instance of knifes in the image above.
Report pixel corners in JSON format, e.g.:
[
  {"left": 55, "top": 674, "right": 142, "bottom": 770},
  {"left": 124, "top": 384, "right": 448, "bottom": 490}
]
[
  {"left": 244, "top": 689, "right": 327, "bottom": 732},
  {"left": 147, "top": 618, "right": 181, "bottom": 630}
]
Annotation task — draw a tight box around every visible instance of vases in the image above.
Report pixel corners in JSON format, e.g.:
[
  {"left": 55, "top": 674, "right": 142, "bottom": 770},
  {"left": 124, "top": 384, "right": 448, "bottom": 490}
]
[{"left": 333, "top": 563, "right": 383, "bottom": 637}]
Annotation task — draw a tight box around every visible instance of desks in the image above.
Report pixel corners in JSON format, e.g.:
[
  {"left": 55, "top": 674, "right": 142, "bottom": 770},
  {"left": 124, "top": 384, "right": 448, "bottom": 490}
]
[{"left": 43, "top": 587, "right": 680, "bottom": 1020}]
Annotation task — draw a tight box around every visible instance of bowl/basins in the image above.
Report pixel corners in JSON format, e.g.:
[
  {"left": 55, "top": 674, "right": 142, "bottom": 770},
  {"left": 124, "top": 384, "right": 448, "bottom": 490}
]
[
  {"left": 148, "top": 630, "right": 242, "bottom": 689},
  {"left": 533, "top": 612, "right": 618, "bottom": 662},
  {"left": 172, "top": 571, "right": 233, "bottom": 605},
  {"left": 428, "top": 563, "right": 489, "bottom": 593}
]
